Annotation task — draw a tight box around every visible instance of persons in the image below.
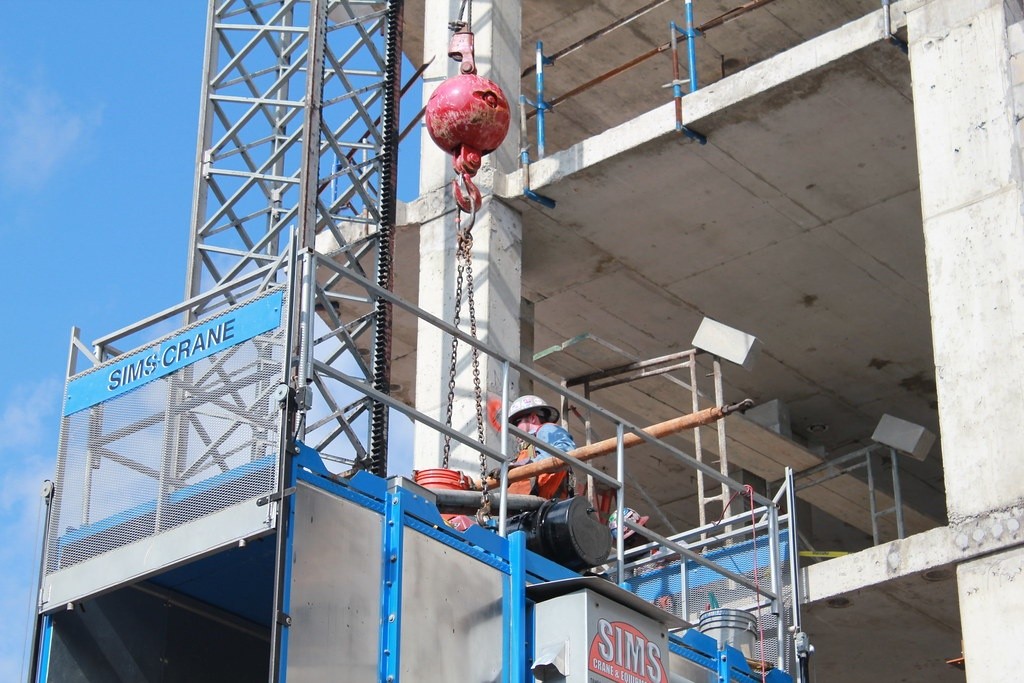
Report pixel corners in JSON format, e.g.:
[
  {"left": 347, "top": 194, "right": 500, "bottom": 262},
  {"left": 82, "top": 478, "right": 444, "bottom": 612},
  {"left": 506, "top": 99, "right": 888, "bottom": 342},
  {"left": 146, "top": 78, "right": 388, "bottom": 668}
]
[
  {"left": 611, "top": 507, "right": 664, "bottom": 563},
  {"left": 484, "top": 394, "right": 578, "bottom": 500}
]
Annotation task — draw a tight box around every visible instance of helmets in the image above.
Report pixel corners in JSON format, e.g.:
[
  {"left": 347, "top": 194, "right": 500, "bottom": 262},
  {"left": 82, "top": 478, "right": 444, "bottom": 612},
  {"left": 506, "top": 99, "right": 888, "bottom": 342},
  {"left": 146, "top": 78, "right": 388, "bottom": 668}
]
[
  {"left": 609, "top": 508, "right": 650, "bottom": 542},
  {"left": 509, "top": 394, "right": 560, "bottom": 423}
]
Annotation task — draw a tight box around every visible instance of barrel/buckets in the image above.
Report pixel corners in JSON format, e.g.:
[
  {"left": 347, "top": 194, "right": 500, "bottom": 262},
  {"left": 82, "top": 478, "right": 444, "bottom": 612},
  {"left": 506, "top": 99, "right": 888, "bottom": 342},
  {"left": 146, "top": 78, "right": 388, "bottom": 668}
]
[
  {"left": 698, "top": 608, "right": 757, "bottom": 659},
  {"left": 413, "top": 466, "right": 469, "bottom": 520}
]
